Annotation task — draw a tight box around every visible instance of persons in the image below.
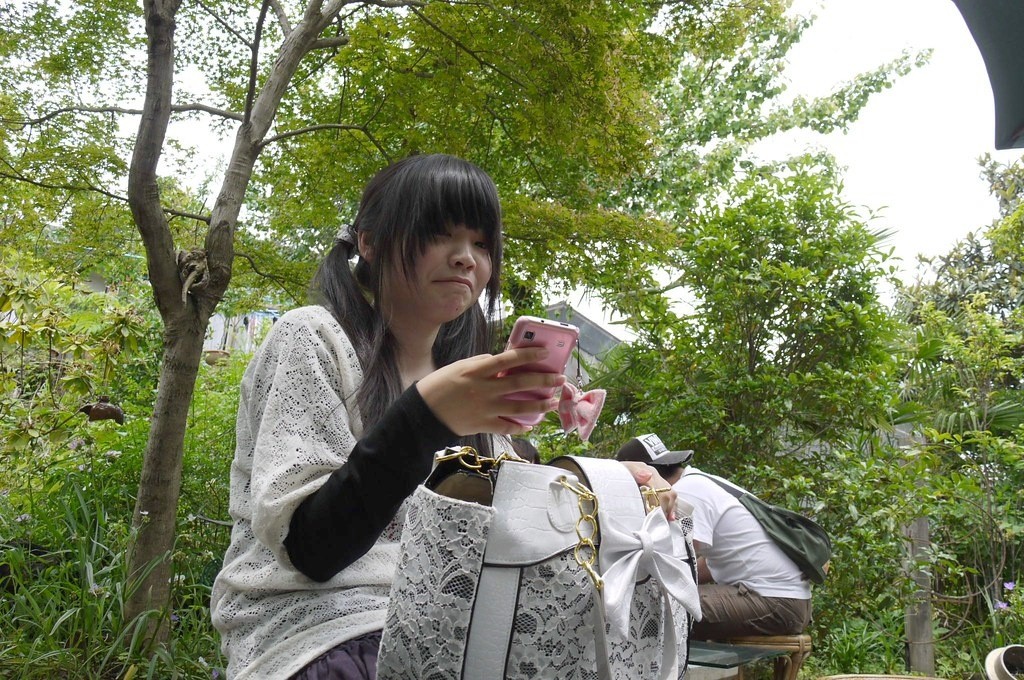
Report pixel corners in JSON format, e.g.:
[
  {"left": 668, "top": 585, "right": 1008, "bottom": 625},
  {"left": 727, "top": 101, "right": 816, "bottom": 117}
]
[
  {"left": 209, "top": 153, "right": 678, "bottom": 680},
  {"left": 616, "top": 440, "right": 811, "bottom": 635}
]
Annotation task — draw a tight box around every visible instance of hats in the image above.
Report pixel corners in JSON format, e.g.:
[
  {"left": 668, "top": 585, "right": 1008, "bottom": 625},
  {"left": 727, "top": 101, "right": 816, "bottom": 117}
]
[{"left": 615, "top": 432, "right": 694, "bottom": 467}]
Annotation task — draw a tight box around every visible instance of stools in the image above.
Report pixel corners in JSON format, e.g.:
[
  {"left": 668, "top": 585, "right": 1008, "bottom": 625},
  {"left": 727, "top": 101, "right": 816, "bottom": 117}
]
[{"left": 713, "top": 634, "right": 813, "bottom": 679}]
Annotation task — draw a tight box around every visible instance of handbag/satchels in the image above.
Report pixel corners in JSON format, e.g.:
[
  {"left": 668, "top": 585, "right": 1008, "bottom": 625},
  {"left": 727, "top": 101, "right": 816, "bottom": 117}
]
[
  {"left": 739, "top": 491, "right": 833, "bottom": 586},
  {"left": 371, "top": 443, "right": 702, "bottom": 680}
]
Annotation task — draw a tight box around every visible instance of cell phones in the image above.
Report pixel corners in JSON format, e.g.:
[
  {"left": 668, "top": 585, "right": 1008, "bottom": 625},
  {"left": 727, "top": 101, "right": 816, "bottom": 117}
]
[{"left": 493, "top": 315, "right": 580, "bottom": 425}]
